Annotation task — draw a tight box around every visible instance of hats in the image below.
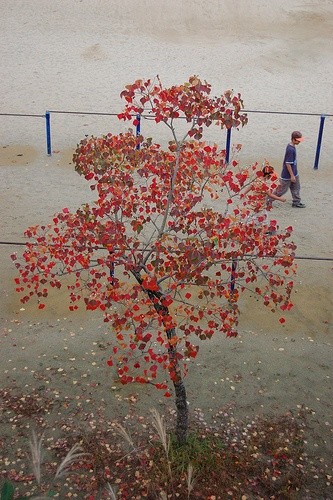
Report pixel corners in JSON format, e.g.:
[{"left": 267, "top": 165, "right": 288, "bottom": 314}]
[
  {"left": 262, "top": 166, "right": 277, "bottom": 177},
  {"left": 291, "top": 130, "right": 304, "bottom": 141}
]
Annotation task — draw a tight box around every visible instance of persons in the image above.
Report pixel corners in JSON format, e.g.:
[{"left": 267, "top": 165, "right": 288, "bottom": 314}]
[
  {"left": 238, "top": 165, "right": 287, "bottom": 238},
  {"left": 265, "top": 131, "right": 307, "bottom": 209}
]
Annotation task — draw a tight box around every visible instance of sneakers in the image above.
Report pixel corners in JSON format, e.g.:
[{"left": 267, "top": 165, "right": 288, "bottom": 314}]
[
  {"left": 292, "top": 202, "right": 307, "bottom": 209},
  {"left": 265, "top": 198, "right": 279, "bottom": 207}
]
